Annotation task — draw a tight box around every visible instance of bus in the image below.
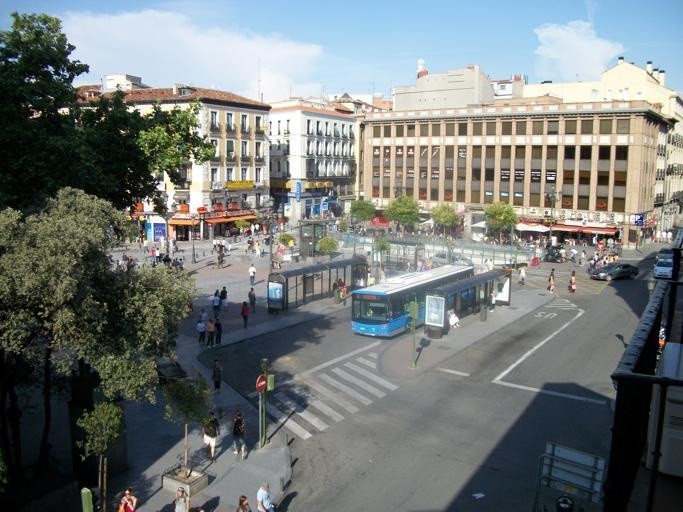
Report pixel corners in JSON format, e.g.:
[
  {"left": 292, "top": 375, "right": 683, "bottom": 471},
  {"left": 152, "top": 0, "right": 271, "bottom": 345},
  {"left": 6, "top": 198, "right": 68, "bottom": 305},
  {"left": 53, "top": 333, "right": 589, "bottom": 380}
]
[{"left": 351, "top": 264, "right": 474, "bottom": 338}]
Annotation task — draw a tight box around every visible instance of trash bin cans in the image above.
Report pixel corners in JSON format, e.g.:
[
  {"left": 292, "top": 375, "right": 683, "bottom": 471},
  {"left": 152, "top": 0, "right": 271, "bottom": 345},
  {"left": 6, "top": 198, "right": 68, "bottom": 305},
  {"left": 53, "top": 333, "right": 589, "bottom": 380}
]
[
  {"left": 480, "top": 304, "right": 487, "bottom": 321},
  {"left": 233, "top": 235, "right": 236, "bottom": 243},
  {"left": 555, "top": 496, "right": 576, "bottom": 511},
  {"left": 334, "top": 288, "right": 340, "bottom": 304}
]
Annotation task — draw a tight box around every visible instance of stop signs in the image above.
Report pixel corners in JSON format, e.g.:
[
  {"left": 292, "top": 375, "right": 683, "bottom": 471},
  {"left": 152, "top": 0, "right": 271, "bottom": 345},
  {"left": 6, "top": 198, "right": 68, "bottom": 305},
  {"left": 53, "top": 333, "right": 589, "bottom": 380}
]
[{"left": 254, "top": 376, "right": 266, "bottom": 391}]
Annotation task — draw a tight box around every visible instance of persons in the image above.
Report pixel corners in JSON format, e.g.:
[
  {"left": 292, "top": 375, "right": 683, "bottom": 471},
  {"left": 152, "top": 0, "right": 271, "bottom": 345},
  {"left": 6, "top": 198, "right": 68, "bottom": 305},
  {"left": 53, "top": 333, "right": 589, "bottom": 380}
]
[
  {"left": 650, "top": 228, "right": 672, "bottom": 243},
  {"left": 229, "top": 410, "right": 246, "bottom": 460},
  {"left": 256, "top": 481, "right": 274, "bottom": 512},
  {"left": 114, "top": 224, "right": 277, "bottom": 349},
  {"left": 491, "top": 286, "right": 496, "bottom": 309},
  {"left": 212, "top": 360, "right": 222, "bottom": 393},
  {"left": 200, "top": 412, "right": 220, "bottom": 463},
  {"left": 447, "top": 308, "right": 460, "bottom": 328},
  {"left": 567, "top": 270, "right": 575, "bottom": 293},
  {"left": 488, "top": 291, "right": 493, "bottom": 313},
  {"left": 547, "top": 268, "right": 555, "bottom": 293},
  {"left": 236, "top": 495, "right": 251, "bottom": 512},
  {"left": 514, "top": 234, "right": 621, "bottom": 284},
  {"left": 329, "top": 224, "right": 513, "bottom": 305},
  {"left": 173, "top": 487, "right": 189, "bottom": 512},
  {"left": 118, "top": 488, "right": 137, "bottom": 512}
]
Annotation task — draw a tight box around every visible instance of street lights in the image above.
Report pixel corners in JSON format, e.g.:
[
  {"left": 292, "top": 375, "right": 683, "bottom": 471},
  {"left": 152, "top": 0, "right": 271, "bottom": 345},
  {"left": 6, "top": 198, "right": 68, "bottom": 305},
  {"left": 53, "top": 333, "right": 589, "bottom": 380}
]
[
  {"left": 261, "top": 357, "right": 271, "bottom": 446},
  {"left": 163, "top": 192, "right": 170, "bottom": 239},
  {"left": 265, "top": 216, "right": 277, "bottom": 275},
  {"left": 658, "top": 193, "right": 673, "bottom": 243},
  {"left": 545, "top": 186, "right": 563, "bottom": 248},
  {"left": 191, "top": 212, "right": 197, "bottom": 263}
]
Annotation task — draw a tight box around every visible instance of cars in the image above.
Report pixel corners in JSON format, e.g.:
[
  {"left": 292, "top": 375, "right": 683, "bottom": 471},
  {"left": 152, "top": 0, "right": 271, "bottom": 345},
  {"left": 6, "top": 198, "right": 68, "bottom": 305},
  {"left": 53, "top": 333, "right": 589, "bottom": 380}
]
[
  {"left": 652, "top": 259, "right": 674, "bottom": 280},
  {"left": 591, "top": 262, "right": 639, "bottom": 281},
  {"left": 430, "top": 251, "right": 472, "bottom": 269},
  {"left": 655, "top": 248, "right": 674, "bottom": 261}
]
